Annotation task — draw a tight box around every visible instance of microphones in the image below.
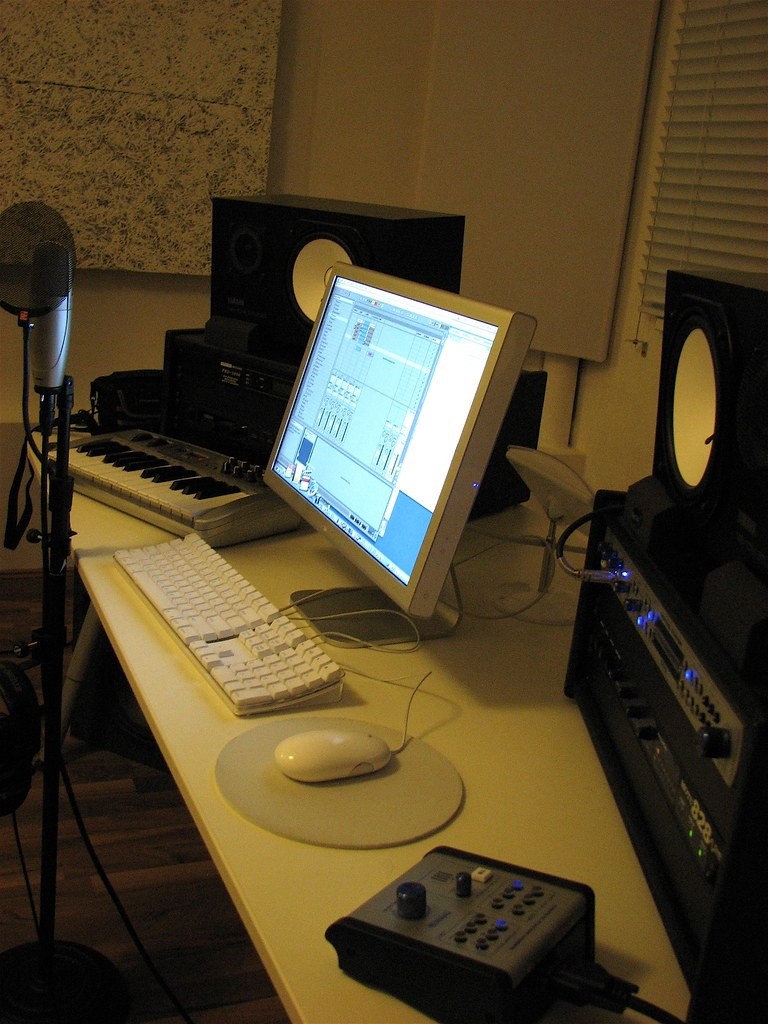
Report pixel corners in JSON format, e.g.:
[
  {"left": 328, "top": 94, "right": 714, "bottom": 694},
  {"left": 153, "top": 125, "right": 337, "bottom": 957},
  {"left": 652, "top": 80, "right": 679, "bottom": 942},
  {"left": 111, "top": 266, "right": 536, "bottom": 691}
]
[{"left": 28, "top": 242, "right": 76, "bottom": 437}]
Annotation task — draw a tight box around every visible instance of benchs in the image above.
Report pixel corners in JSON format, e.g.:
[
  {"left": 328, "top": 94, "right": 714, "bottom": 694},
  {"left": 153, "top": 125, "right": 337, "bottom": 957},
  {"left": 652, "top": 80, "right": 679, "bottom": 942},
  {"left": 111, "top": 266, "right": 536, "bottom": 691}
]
[{"left": 0, "top": 269, "right": 214, "bottom": 579}]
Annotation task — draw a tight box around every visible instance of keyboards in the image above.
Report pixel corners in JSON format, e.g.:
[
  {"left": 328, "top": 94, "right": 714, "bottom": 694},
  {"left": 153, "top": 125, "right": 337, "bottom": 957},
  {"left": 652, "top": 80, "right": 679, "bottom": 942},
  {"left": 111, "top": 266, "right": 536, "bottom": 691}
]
[{"left": 111, "top": 534, "right": 347, "bottom": 717}]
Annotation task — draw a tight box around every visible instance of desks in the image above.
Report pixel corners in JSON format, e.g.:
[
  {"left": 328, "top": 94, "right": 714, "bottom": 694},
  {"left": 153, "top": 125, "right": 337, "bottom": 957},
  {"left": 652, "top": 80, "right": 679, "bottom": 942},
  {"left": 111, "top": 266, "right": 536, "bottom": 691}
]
[{"left": 27, "top": 430, "right": 697, "bottom": 1024}]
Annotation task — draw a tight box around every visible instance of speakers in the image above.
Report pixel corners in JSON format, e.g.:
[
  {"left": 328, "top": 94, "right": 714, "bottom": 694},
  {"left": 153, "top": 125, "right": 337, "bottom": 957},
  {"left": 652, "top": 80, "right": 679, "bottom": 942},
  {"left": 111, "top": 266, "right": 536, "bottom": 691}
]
[
  {"left": 654, "top": 268, "right": 768, "bottom": 585},
  {"left": 209, "top": 193, "right": 466, "bottom": 333}
]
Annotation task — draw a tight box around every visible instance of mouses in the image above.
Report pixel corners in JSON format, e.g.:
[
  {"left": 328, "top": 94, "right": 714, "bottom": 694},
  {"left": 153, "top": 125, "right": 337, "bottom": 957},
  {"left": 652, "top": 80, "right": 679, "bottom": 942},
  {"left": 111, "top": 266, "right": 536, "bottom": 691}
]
[{"left": 274, "top": 730, "right": 390, "bottom": 782}]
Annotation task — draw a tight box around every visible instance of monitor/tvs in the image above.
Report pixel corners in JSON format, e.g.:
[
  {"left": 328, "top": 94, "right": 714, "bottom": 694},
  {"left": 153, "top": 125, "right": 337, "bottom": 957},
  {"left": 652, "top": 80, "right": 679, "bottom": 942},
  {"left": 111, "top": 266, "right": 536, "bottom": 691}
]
[{"left": 261, "top": 263, "right": 540, "bottom": 649}]
